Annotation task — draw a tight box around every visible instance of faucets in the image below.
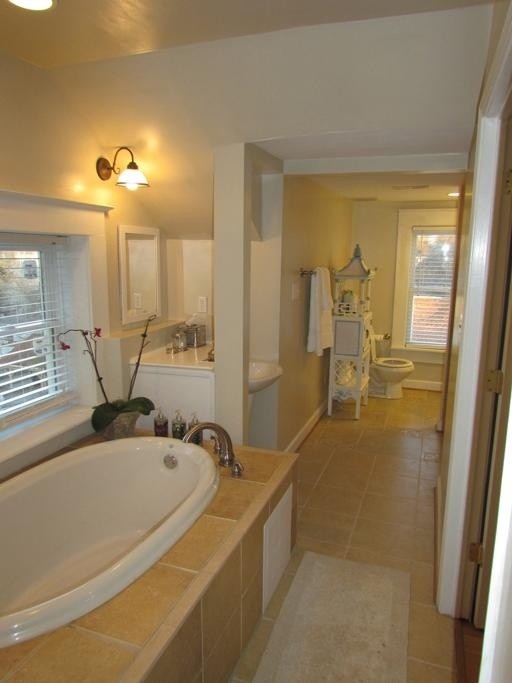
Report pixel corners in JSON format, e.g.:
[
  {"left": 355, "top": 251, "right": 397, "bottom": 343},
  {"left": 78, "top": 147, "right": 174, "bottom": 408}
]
[
  {"left": 207, "top": 348, "right": 214, "bottom": 361},
  {"left": 183, "top": 422, "right": 235, "bottom": 467}
]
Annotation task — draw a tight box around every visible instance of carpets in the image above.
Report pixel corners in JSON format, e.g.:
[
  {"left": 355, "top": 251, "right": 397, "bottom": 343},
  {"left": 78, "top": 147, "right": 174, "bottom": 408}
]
[{"left": 249, "top": 551, "right": 411, "bottom": 683}]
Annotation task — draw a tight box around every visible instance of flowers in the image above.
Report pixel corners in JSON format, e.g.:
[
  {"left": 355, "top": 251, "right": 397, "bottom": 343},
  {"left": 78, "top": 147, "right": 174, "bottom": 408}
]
[{"left": 56, "top": 312, "right": 158, "bottom": 433}]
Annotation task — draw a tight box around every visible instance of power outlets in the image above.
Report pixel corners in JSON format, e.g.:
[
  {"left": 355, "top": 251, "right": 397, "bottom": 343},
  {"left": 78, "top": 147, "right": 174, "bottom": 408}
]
[
  {"left": 198, "top": 297, "right": 208, "bottom": 313},
  {"left": 134, "top": 294, "right": 143, "bottom": 307}
]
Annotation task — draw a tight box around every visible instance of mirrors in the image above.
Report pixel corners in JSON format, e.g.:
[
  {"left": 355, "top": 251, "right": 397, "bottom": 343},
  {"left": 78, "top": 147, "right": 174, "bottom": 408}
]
[{"left": 118, "top": 224, "right": 161, "bottom": 326}]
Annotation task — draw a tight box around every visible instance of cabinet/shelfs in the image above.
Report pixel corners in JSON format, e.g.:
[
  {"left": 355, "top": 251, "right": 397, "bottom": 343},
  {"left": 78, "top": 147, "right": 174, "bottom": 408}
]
[{"left": 327, "top": 312, "right": 371, "bottom": 421}]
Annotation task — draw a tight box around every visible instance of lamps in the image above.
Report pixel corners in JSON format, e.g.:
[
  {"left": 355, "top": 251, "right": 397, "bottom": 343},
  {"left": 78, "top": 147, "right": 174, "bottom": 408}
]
[{"left": 96, "top": 146, "right": 148, "bottom": 191}]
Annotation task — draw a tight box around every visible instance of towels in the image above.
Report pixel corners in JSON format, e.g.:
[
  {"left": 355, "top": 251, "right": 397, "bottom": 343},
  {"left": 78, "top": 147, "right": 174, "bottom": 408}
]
[{"left": 307, "top": 267, "right": 335, "bottom": 357}]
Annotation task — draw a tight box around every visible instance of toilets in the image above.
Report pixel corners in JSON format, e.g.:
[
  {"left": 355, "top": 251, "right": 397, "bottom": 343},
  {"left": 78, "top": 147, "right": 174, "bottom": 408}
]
[{"left": 368, "top": 325, "right": 415, "bottom": 399}]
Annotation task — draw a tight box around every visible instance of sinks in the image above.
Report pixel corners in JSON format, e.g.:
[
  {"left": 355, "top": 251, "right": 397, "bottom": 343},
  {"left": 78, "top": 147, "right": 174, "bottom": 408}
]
[{"left": 247, "top": 361, "right": 284, "bottom": 394}]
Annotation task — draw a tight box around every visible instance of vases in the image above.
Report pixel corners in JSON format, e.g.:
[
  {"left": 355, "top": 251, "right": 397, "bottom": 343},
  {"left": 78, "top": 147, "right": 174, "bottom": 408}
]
[{"left": 103, "top": 411, "right": 140, "bottom": 439}]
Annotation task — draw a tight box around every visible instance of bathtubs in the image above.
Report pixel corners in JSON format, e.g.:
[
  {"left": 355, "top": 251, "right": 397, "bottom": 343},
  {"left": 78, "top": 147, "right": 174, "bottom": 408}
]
[{"left": 0, "top": 436, "right": 221, "bottom": 649}]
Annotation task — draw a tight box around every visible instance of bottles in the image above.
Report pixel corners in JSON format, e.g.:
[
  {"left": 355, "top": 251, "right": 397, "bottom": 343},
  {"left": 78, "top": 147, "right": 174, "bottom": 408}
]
[
  {"left": 174, "top": 333, "right": 187, "bottom": 351},
  {"left": 166, "top": 337, "right": 175, "bottom": 353}
]
